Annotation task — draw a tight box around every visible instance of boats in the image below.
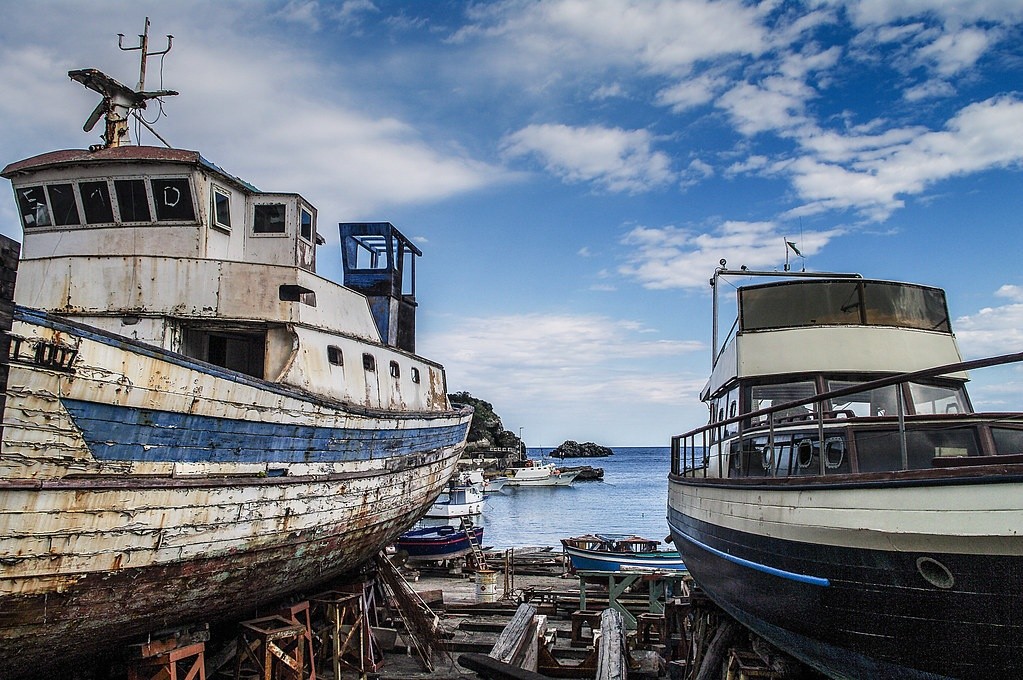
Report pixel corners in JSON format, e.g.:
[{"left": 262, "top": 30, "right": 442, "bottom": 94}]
[
  {"left": 393, "top": 520, "right": 484, "bottom": 557},
  {"left": 503, "top": 459, "right": 582, "bottom": 486},
  {"left": 666, "top": 237, "right": 1023, "bottom": 679},
  {"left": 423, "top": 467, "right": 489, "bottom": 519},
  {"left": 442, "top": 468, "right": 508, "bottom": 493},
  {"left": 565, "top": 545, "right": 689, "bottom": 570},
  {"left": 0, "top": 19, "right": 474, "bottom": 679}
]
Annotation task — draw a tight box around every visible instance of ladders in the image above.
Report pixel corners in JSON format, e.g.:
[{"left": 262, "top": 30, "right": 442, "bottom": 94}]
[{"left": 460, "top": 516, "right": 489, "bottom": 570}]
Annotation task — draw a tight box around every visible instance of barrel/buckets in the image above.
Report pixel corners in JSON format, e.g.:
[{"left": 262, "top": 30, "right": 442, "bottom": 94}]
[{"left": 475, "top": 570, "right": 497, "bottom": 603}]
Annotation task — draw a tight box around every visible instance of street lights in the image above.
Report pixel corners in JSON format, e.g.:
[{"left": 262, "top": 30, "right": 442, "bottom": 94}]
[{"left": 520, "top": 427, "right": 524, "bottom": 462}]
